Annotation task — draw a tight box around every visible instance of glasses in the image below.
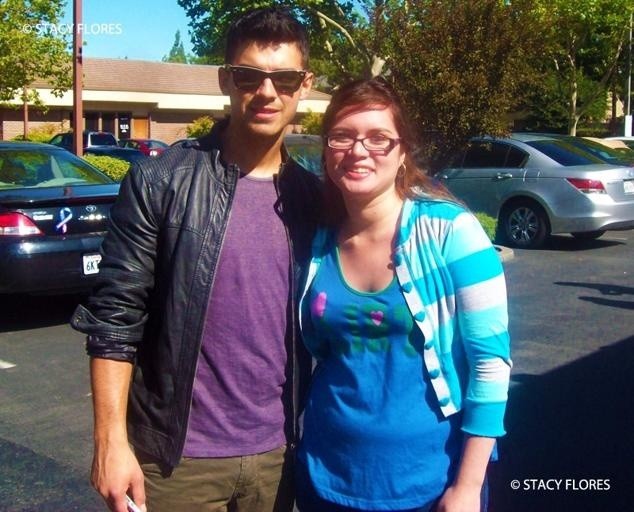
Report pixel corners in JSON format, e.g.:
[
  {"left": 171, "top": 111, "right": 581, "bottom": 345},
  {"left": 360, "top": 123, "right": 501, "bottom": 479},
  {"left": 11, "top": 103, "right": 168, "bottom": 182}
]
[
  {"left": 223, "top": 63, "right": 307, "bottom": 95},
  {"left": 321, "top": 133, "right": 403, "bottom": 154}
]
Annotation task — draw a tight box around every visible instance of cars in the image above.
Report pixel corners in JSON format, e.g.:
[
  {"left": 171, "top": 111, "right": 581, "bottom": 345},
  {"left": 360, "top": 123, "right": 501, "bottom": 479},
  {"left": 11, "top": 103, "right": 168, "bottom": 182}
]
[
  {"left": 42, "top": 131, "right": 169, "bottom": 162},
  {"left": 1, "top": 142, "right": 120, "bottom": 297},
  {"left": 410, "top": 132, "right": 634, "bottom": 249},
  {"left": 281, "top": 134, "right": 329, "bottom": 184}
]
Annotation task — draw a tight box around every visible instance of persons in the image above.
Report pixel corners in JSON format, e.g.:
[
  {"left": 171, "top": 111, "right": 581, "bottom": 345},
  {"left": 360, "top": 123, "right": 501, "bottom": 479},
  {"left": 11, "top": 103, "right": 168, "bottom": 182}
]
[
  {"left": 293, "top": 74, "right": 514, "bottom": 512},
  {"left": 70, "top": 7, "right": 324, "bottom": 511}
]
